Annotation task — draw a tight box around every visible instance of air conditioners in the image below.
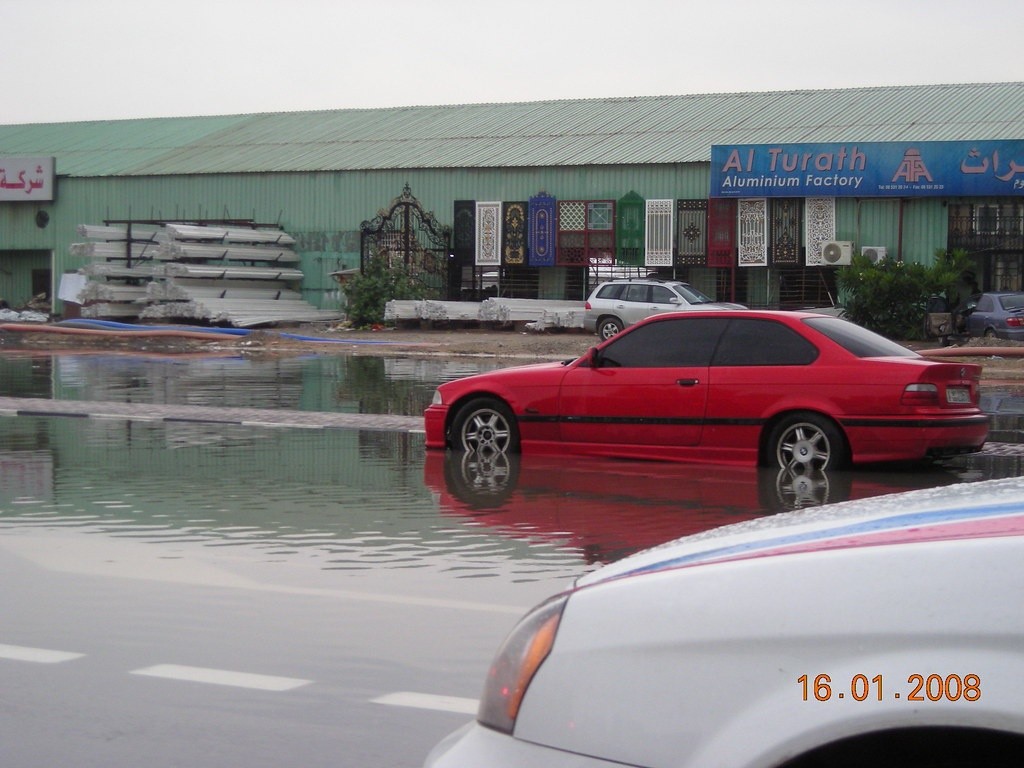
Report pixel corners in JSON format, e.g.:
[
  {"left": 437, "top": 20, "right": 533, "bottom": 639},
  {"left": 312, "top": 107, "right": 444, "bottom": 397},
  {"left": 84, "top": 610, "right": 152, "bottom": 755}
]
[
  {"left": 822, "top": 241, "right": 855, "bottom": 266},
  {"left": 861, "top": 247, "right": 886, "bottom": 267}
]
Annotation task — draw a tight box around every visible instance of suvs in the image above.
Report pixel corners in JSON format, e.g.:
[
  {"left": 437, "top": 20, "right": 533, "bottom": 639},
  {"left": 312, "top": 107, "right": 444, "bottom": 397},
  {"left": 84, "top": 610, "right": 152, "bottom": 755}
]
[{"left": 582, "top": 276, "right": 752, "bottom": 344}]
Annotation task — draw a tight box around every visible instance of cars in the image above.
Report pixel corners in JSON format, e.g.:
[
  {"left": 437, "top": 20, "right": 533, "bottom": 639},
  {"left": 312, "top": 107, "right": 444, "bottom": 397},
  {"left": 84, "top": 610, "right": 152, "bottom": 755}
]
[
  {"left": 968, "top": 290, "right": 1024, "bottom": 343},
  {"left": 414, "top": 474, "right": 1023, "bottom": 768},
  {"left": 422, "top": 444, "right": 965, "bottom": 549},
  {"left": 418, "top": 309, "right": 993, "bottom": 470}
]
